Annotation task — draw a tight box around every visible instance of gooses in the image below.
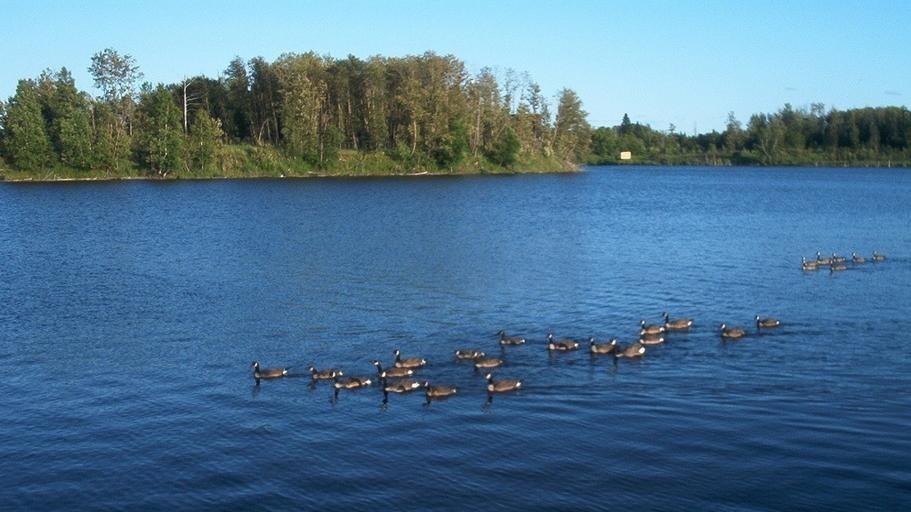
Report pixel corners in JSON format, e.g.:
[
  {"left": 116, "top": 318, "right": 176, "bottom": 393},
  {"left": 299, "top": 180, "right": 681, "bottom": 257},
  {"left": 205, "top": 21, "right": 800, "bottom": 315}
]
[
  {"left": 453, "top": 348, "right": 525, "bottom": 395},
  {"left": 545, "top": 333, "right": 579, "bottom": 351},
  {"left": 248, "top": 360, "right": 294, "bottom": 379},
  {"left": 751, "top": 315, "right": 781, "bottom": 328},
  {"left": 718, "top": 323, "right": 746, "bottom": 339},
  {"left": 588, "top": 335, "right": 617, "bottom": 353},
  {"left": 307, "top": 347, "right": 431, "bottom": 396},
  {"left": 618, "top": 309, "right": 696, "bottom": 359},
  {"left": 801, "top": 252, "right": 888, "bottom": 274},
  {"left": 420, "top": 380, "right": 456, "bottom": 400},
  {"left": 494, "top": 329, "right": 526, "bottom": 345}
]
